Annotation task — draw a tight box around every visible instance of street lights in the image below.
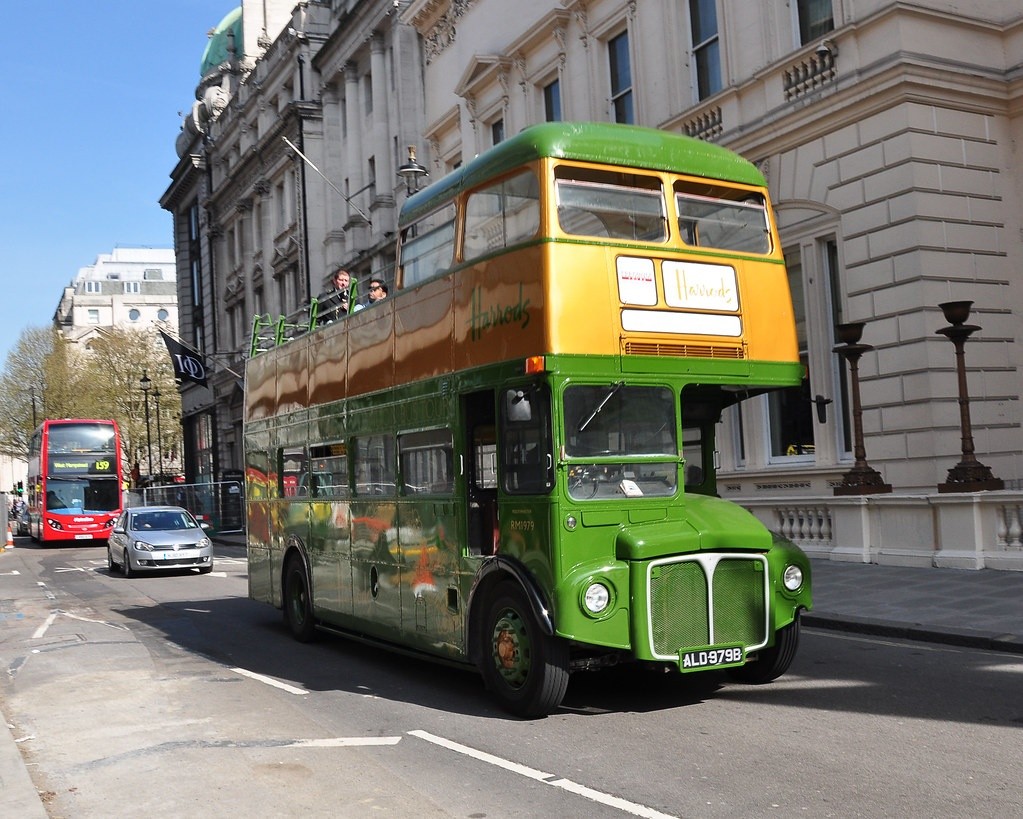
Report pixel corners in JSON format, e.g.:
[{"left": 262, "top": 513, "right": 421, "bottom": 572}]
[
  {"left": 151, "top": 385, "right": 165, "bottom": 490},
  {"left": 140, "top": 368, "right": 157, "bottom": 499}
]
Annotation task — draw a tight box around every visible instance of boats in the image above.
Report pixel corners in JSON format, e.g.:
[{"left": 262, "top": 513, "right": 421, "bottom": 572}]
[{"left": 17, "top": 481, "right": 24, "bottom": 495}]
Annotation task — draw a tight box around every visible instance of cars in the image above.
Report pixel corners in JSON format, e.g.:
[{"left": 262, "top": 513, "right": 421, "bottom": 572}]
[
  {"left": 15, "top": 505, "right": 31, "bottom": 536},
  {"left": 107, "top": 505, "right": 214, "bottom": 577}
]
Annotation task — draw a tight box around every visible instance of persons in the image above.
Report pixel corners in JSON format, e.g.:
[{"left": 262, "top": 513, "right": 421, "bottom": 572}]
[
  {"left": 528, "top": 427, "right": 587, "bottom": 486},
  {"left": 364, "top": 280, "right": 388, "bottom": 308},
  {"left": 12, "top": 496, "right": 31, "bottom": 537},
  {"left": 314, "top": 269, "right": 361, "bottom": 326},
  {"left": 176, "top": 486, "right": 203, "bottom": 517}
]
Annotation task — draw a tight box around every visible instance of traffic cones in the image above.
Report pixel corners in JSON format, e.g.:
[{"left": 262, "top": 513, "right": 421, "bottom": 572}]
[{"left": 4, "top": 524, "right": 15, "bottom": 550}]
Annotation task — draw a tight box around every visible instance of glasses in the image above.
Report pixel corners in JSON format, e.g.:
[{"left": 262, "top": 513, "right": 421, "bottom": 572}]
[{"left": 368, "top": 286, "right": 382, "bottom": 292}]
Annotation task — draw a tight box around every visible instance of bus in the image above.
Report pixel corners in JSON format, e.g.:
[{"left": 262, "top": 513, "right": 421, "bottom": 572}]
[
  {"left": 27, "top": 417, "right": 124, "bottom": 548},
  {"left": 242, "top": 123, "right": 819, "bottom": 718},
  {"left": 244, "top": 413, "right": 545, "bottom": 632}
]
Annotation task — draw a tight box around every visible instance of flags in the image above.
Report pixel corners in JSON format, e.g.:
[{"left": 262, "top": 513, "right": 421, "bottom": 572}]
[{"left": 158, "top": 328, "right": 209, "bottom": 390}]
